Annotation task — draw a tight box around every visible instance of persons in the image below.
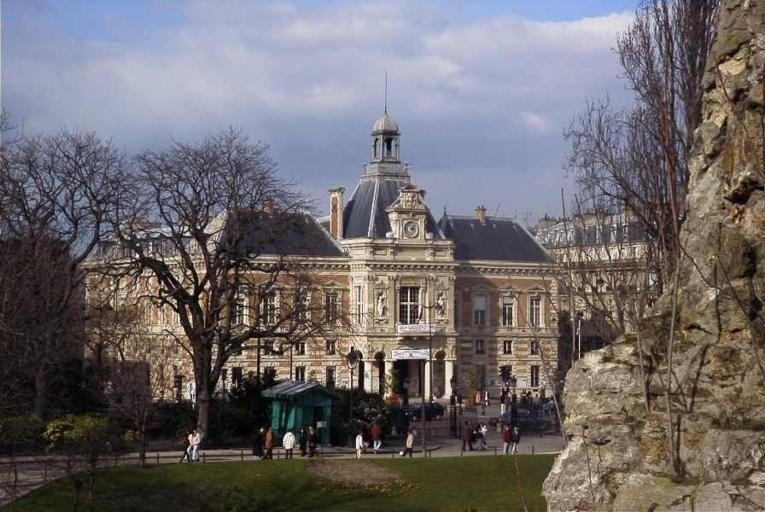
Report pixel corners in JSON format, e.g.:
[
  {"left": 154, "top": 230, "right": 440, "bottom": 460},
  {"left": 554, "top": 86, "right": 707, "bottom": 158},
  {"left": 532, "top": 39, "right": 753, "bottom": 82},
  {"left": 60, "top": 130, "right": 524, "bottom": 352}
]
[
  {"left": 171, "top": 422, "right": 319, "bottom": 464},
  {"left": 351, "top": 382, "right": 563, "bottom": 457}
]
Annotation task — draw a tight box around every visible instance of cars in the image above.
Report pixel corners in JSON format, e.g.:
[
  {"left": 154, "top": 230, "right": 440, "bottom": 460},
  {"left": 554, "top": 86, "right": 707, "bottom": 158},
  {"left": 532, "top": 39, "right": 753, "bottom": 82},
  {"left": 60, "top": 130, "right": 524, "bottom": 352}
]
[
  {"left": 498, "top": 411, "right": 553, "bottom": 434},
  {"left": 405, "top": 401, "right": 444, "bottom": 422}
]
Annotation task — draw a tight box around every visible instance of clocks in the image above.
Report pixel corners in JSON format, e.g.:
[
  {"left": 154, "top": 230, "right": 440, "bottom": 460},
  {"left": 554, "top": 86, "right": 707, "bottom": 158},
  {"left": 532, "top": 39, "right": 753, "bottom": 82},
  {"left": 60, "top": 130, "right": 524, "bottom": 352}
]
[{"left": 402, "top": 220, "right": 419, "bottom": 238}]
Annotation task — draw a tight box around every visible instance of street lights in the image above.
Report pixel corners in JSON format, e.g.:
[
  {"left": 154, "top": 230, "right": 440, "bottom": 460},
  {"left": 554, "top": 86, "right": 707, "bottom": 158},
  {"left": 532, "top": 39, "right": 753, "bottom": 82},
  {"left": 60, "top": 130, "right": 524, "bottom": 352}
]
[
  {"left": 411, "top": 274, "right": 444, "bottom": 402},
  {"left": 346, "top": 345, "right": 357, "bottom": 426}
]
